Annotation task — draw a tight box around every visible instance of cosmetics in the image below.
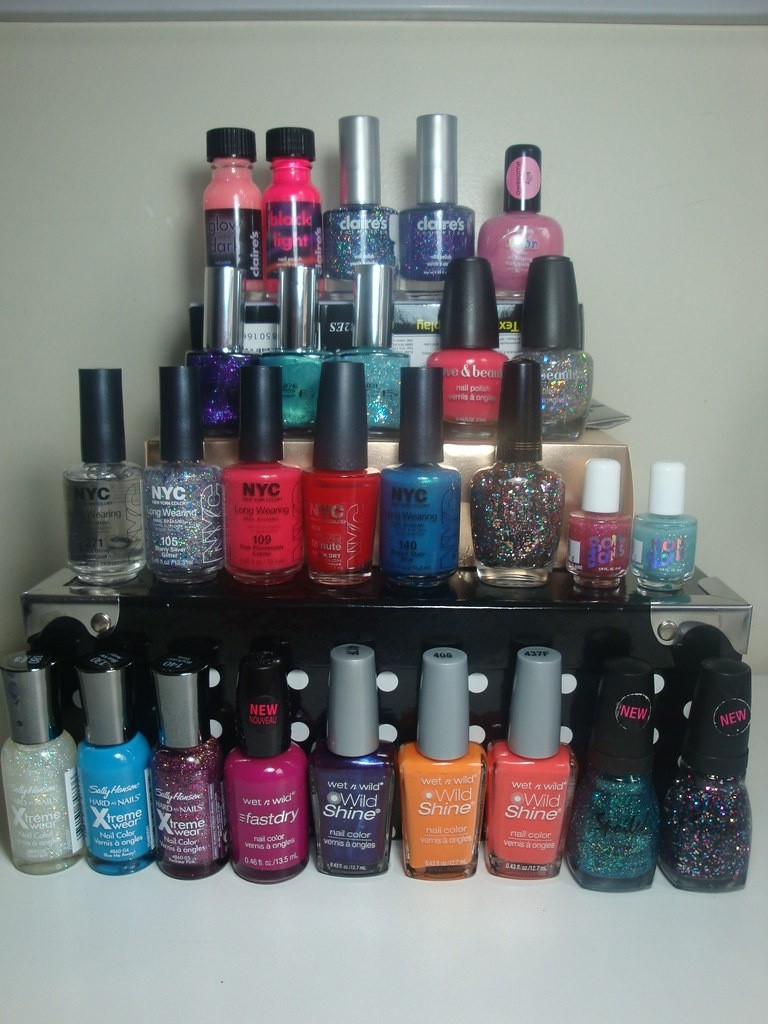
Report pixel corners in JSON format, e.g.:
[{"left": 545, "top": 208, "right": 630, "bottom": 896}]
[{"left": 0, "top": 110, "right": 751, "bottom": 891}]
